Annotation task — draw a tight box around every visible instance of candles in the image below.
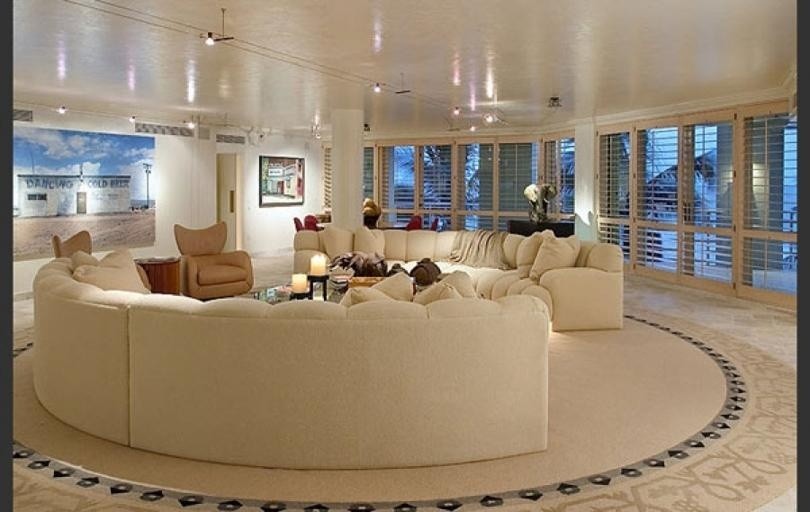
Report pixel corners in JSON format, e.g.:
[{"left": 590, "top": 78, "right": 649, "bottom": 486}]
[
  {"left": 310, "top": 253, "right": 326, "bottom": 276},
  {"left": 291, "top": 273, "right": 307, "bottom": 294}
]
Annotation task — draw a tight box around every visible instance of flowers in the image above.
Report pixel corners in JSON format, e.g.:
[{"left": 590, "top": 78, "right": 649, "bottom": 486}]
[{"left": 523, "top": 182, "right": 557, "bottom": 205}]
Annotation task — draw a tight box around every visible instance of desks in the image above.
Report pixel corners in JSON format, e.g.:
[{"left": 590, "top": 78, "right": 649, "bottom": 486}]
[
  {"left": 136, "top": 256, "right": 181, "bottom": 295},
  {"left": 314, "top": 221, "right": 409, "bottom": 230},
  {"left": 347, "top": 277, "right": 385, "bottom": 289},
  {"left": 507, "top": 218, "right": 574, "bottom": 238}
]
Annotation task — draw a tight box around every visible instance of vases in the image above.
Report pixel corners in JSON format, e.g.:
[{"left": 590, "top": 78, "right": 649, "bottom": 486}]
[{"left": 529, "top": 202, "right": 548, "bottom": 224}]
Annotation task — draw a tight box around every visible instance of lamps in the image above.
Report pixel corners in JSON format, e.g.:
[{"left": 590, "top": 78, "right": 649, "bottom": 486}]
[
  {"left": 374, "top": 82, "right": 381, "bottom": 93},
  {"left": 363, "top": 123, "right": 371, "bottom": 138},
  {"left": 205, "top": 32, "right": 218, "bottom": 44},
  {"left": 454, "top": 107, "right": 462, "bottom": 115}
]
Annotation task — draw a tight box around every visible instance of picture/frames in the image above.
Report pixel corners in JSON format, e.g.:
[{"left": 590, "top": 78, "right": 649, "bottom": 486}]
[{"left": 260, "top": 156, "right": 305, "bottom": 207}]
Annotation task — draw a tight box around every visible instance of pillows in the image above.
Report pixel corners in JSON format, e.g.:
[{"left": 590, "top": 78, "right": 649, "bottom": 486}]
[
  {"left": 295, "top": 229, "right": 581, "bottom": 285},
  {"left": 338, "top": 269, "right": 478, "bottom": 311},
  {"left": 69, "top": 248, "right": 154, "bottom": 294}
]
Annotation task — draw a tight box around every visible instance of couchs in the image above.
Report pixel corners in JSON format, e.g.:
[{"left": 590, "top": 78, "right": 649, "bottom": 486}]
[
  {"left": 294, "top": 229, "right": 624, "bottom": 333},
  {"left": 31, "top": 256, "right": 550, "bottom": 470}
]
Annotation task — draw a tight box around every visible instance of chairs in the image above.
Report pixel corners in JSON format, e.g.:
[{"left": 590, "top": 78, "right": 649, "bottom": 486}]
[
  {"left": 431, "top": 218, "right": 438, "bottom": 231},
  {"left": 405, "top": 214, "right": 421, "bottom": 230},
  {"left": 174, "top": 221, "right": 254, "bottom": 301},
  {"left": 304, "top": 216, "right": 318, "bottom": 231},
  {"left": 51, "top": 230, "right": 92, "bottom": 259},
  {"left": 294, "top": 218, "right": 305, "bottom": 232}
]
